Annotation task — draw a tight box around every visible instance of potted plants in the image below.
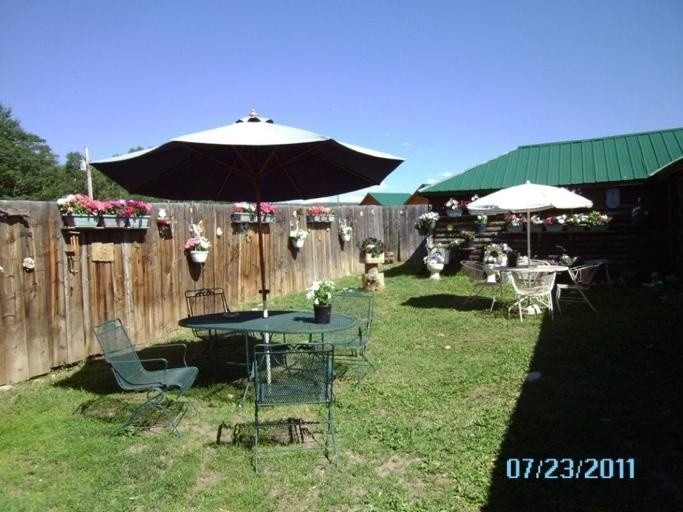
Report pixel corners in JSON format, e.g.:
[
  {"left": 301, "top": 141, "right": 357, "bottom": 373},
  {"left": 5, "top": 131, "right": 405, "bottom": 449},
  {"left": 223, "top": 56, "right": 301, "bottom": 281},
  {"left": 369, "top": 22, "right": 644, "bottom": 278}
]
[{"left": 358, "top": 236, "right": 385, "bottom": 258}]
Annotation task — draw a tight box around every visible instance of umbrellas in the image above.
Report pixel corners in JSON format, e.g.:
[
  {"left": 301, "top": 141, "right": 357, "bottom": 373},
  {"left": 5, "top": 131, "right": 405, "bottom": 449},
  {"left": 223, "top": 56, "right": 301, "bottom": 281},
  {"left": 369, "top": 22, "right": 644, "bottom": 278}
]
[
  {"left": 87, "top": 109, "right": 406, "bottom": 383},
  {"left": 468, "top": 179, "right": 594, "bottom": 302}
]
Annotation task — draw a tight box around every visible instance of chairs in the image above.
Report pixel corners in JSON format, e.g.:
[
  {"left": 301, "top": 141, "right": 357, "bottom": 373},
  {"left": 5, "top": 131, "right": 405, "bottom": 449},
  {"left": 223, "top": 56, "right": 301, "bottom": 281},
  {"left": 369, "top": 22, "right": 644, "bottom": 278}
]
[
  {"left": 246, "top": 341, "right": 344, "bottom": 476},
  {"left": 459, "top": 260, "right": 602, "bottom": 324},
  {"left": 90, "top": 317, "right": 202, "bottom": 440},
  {"left": 183, "top": 288, "right": 272, "bottom": 382},
  {"left": 304, "top": 287, "right": 376, "bottom": 388}
]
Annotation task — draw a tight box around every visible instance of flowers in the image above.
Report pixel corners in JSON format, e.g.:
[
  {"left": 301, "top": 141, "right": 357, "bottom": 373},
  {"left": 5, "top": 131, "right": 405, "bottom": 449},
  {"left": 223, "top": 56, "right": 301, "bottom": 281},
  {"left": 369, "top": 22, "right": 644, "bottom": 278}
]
[
  {"left": 306, "top": 278, "right": 336, "bottom": 307},
  {"left": 416, "top": 196, "right": 614, "bottom": 285}
]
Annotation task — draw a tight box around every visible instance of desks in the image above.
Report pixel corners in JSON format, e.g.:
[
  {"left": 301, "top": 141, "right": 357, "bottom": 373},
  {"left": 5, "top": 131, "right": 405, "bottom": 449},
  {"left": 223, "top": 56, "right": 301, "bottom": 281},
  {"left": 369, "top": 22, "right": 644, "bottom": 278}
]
[{"left": 177, "top": 308, "right": 358, "bottom": 409}]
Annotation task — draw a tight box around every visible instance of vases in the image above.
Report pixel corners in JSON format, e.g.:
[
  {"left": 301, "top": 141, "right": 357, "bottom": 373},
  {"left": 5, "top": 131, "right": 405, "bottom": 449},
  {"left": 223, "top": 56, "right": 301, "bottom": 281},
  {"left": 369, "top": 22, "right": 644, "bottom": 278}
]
[{"left": 312, "top": 304, "right": 332, "bottom": 324}]
